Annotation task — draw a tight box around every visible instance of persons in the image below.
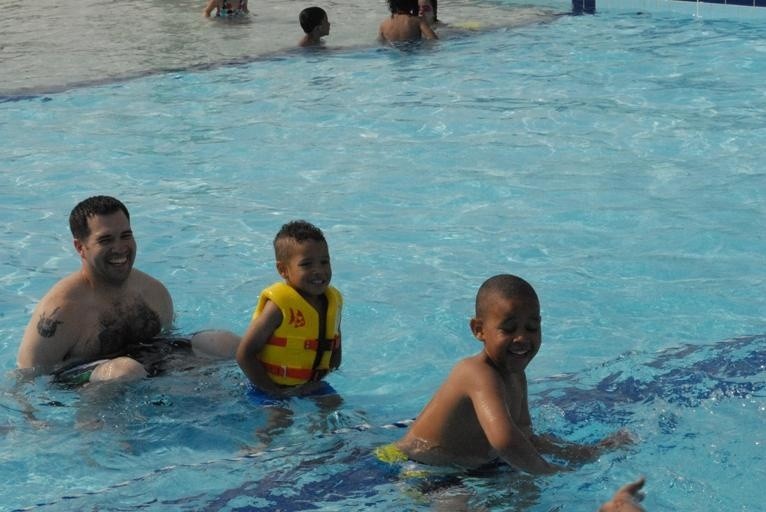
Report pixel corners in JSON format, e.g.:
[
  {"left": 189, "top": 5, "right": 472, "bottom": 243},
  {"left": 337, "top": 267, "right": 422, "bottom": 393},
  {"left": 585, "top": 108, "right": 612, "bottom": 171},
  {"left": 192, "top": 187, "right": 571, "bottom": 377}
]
[
  {"left": 571, "top": 1, "right": 597, "bottom": 16},
  {"left": 12, "top": 195, "right": 244, "bottom": 434},
  {"left": 235, "top": 221, "right": 343, "bottom": 455},
  {"left": 394, "top": 275, "right": 579, "bottom": 475},
  {"left": 299, "top": 8, "right": 331, "bottom": 49},
  {"left": 375, "top": 1, "right": 449, "bottom": 44},
  {"left": 204, "top": 1, "right": 258, "bottom": 21}
]
